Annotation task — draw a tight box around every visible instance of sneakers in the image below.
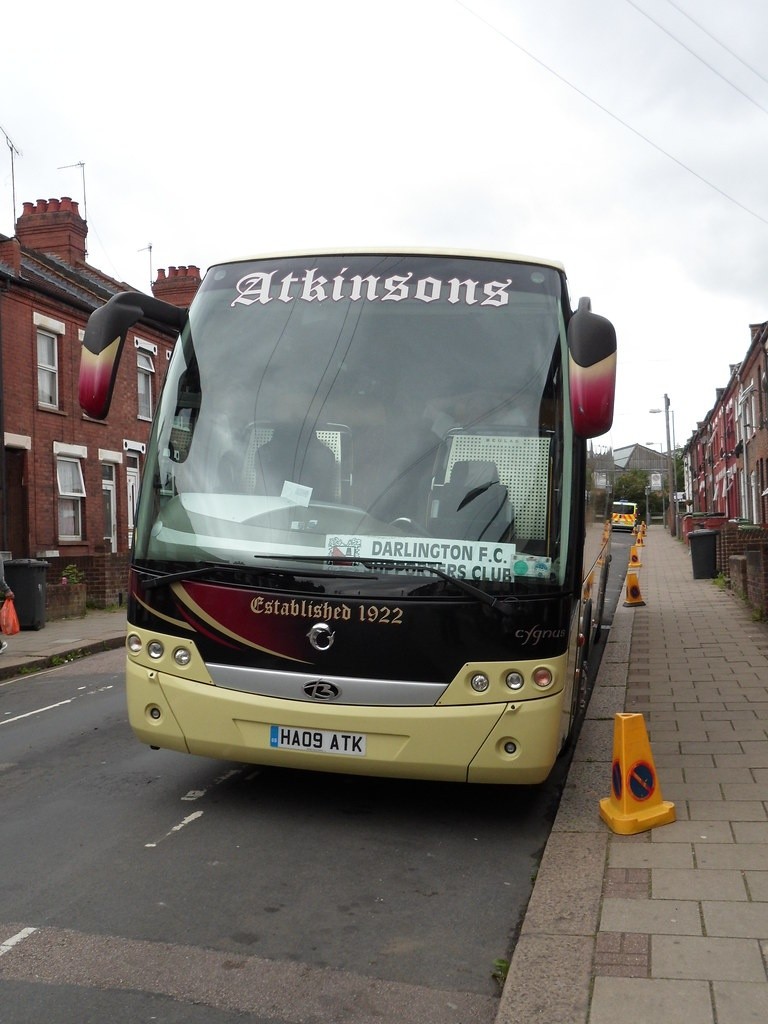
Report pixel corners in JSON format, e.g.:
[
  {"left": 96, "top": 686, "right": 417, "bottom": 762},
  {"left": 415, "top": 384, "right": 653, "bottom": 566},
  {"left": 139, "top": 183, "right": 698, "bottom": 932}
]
[{"left": 0, "top": 642, "right": 7, "bottom": 654}]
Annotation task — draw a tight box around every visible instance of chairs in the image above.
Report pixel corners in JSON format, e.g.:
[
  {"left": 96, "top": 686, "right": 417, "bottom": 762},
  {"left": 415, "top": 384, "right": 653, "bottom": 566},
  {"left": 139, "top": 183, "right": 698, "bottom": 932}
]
[
  {"left": 426, "top": 460, "right": 515, "bottom": 545},
  {"left": 250, "top": 415, "right": 338, "bottom": 503}
]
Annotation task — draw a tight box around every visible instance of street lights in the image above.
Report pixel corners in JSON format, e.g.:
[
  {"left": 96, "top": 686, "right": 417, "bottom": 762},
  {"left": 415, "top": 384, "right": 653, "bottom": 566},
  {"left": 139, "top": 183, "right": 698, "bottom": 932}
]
[
  {"left": 646, "top": 442, "right": 666, "bottom": 529},
  {"left": 649, "top": 409, "right": 679, "bottom": 539}
]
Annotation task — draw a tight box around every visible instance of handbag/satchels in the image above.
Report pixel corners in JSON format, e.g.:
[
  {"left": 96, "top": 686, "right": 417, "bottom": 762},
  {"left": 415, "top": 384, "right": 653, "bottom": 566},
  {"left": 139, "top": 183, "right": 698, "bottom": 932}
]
[{"left": 0, "top": 597, "right": 19, "bottom": 635}]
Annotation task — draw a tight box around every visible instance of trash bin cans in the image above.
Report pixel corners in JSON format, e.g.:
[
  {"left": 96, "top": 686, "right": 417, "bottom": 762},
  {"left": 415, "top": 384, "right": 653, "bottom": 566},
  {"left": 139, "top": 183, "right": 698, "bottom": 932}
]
[
  {"left": 687, "top": 528, "right": 719, "bottom": 579},
  {"left": 2, "top": 558, "right": 49, "bottom": 631}
]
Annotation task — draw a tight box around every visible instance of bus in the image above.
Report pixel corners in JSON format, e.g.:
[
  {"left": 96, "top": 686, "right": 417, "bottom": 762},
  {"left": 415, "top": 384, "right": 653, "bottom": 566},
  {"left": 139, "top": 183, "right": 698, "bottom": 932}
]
[
  {"left": 77, "top": 251, "right": 618, "bottom": 783},
  {"left": 611, "top": 499, "right": 638, "bottom": 532}
]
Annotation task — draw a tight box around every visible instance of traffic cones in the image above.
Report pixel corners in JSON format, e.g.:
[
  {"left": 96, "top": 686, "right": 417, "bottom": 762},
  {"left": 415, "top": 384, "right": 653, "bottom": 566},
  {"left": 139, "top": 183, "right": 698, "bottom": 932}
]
[
  {"left": 597, "top": 714, "right": 676, "bottom": 836},
  {"left": 641, "top": 521, "right": 646, "bottom": 530},
  {"left": 635, "top": 532, "right": 645, "bottom": 547},
  {"left": 639, "top": 523, "right": 647, "bottom": 537},
  {"left": 628, "top": 546, "right": 642, "bottom": 567},
  {"left": 600, "top": 519, "right": 610, "bottom": 545},
  {"left": 630, "top": 526, "right": 639, "bottom": 536},
  {"left": 623, "top": 573, "right": 646, "bottom": 607}
]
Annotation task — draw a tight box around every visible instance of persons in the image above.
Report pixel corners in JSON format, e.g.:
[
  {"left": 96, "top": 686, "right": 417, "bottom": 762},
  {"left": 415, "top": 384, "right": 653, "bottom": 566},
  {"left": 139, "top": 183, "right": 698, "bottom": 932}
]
[{"left": 0, "top": 550, "right": 15, "bottom": 655}]
